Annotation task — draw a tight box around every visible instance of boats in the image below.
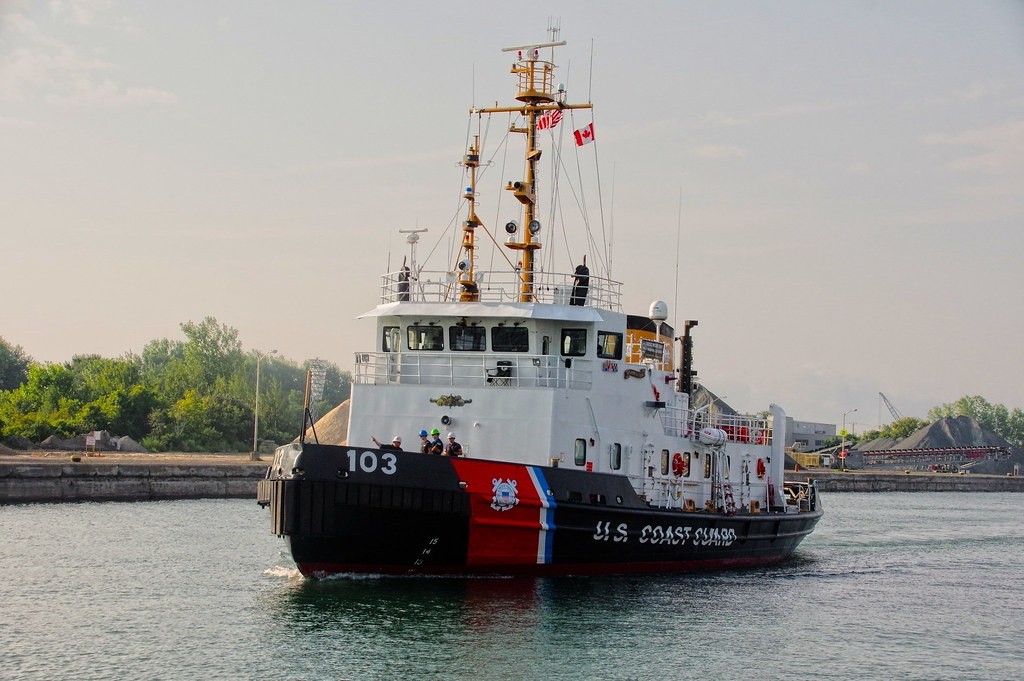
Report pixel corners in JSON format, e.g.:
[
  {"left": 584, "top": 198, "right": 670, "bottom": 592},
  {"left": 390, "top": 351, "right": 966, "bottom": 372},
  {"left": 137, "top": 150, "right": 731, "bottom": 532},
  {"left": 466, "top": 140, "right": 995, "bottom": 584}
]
[{"left": 245, "top": 24, "right": 822, "bottom": 578}]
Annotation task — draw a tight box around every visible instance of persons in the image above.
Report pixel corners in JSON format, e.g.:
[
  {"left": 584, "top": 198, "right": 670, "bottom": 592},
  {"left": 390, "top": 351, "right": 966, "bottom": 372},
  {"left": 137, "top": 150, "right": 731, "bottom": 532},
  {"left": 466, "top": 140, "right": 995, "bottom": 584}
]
[
  {"left": 443, "top": 432, "right": 462, "bottom": 457},
  {"left": 418, "top": 430, "right": 432, "bottom": 454},
  {"left": 370, "top": 433, "right": 403, "bottom": 451},
  {"left": 427, "top": 429, "right": 443, "bottom": 455}
]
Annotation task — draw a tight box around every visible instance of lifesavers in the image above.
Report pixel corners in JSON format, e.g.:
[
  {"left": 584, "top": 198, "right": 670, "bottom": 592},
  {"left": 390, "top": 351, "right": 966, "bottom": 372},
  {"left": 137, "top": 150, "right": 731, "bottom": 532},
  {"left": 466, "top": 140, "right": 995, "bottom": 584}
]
[
  {"left": 757, "top": 458, "right": 766, "bottom": 478},
  {"left": 673, "top": 453, "right": 682, "bottom": 476}
]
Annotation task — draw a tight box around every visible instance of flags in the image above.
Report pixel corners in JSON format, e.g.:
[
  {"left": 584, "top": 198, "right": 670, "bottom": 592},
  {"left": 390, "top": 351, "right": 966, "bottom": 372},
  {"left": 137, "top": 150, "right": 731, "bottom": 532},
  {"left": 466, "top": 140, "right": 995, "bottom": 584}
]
[
  {"left": 573, "top": 123, "right": 595, "bottom": 147},
  {"left": 537, "top": 108, "right": 563, "bottom": 130}
]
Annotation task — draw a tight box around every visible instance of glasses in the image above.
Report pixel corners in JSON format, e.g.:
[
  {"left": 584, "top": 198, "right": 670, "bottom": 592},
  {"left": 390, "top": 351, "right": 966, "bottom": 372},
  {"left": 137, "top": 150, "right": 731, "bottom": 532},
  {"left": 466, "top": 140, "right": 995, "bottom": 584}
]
[
  {"left": 432, "top": 434, "right": 436, "bottom": 436},
  {"left": 420, "top": 435, "right": 423, "bottom": 437}
]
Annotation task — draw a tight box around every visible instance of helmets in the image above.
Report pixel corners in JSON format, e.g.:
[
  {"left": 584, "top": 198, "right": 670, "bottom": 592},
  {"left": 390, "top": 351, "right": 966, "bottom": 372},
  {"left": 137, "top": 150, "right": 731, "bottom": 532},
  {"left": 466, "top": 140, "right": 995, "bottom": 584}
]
[
  {"left": 418, "top": 430, "right": 428, "bottom": 436},
  {"left": 431, "top": 428, "right": 439, "bottom": 434},
  {"left": 393, "top": 436, "right": 402, "bottom": 443},
  {"left": 446, "top": 432, "right": 456, "bottom": 438}
]
